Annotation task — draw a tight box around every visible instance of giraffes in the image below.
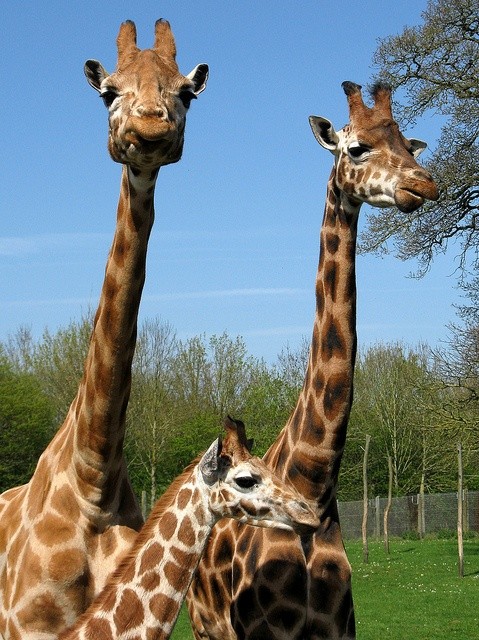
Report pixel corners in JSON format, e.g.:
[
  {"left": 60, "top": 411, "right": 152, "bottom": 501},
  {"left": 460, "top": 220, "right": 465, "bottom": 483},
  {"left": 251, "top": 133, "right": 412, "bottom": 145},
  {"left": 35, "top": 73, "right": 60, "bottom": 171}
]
[
  {"left": 186, "top": 81, "right": 440, "bottom": 640},
  {"left": 55, "top": 414, "right": 322, "bottom": 640},
  {"left": 0, "top": 18, "right": 209, "bottom": 640}
]
